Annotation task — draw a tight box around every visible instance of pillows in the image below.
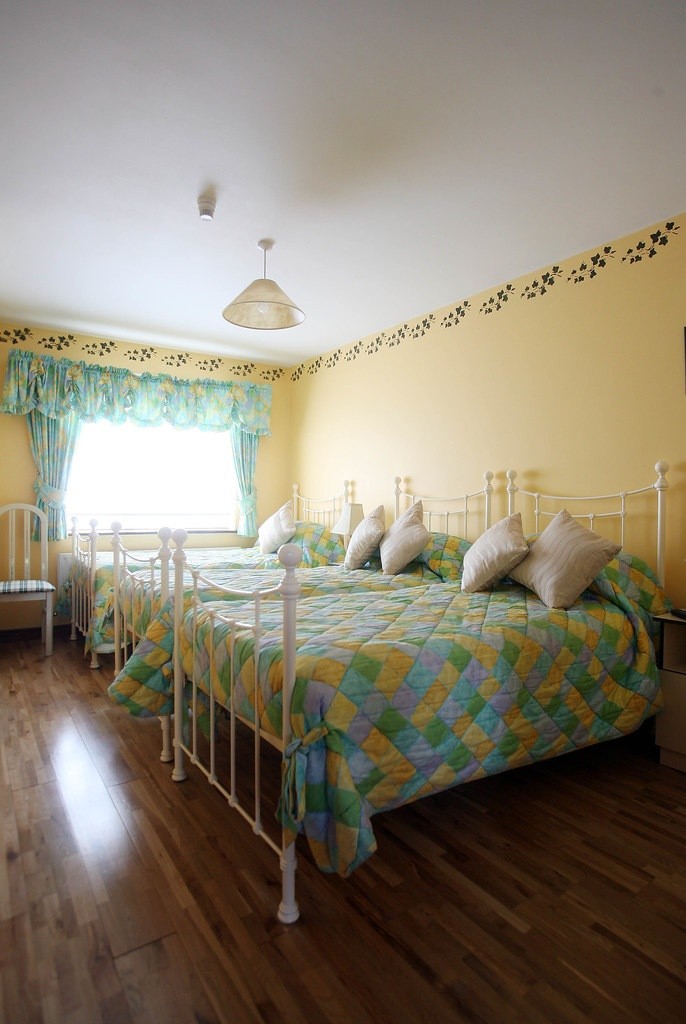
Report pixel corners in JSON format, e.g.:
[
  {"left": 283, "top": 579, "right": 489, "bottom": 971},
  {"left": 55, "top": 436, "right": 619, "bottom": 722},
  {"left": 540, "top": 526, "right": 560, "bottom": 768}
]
[
  {"left": 508, "top": 507, "right": 622, "bottom": 609},
  {"left": 459, "top": 510, "right": 530, "bottom": 593},
  {"left": 258, "top": 499, "right": 297, "bottom": 554},
  {"left": 379, "top": 500, "right": 433, "bottom": 575},
  {"left": 343, "top": 505, "right": 385, "bottom": 570}
]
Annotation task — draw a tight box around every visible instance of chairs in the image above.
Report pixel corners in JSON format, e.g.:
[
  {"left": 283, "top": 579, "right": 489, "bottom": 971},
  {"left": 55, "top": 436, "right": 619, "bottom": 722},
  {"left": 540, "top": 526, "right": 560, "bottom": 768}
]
[{"left": 0, "top": 503, "right": 56, "bottom": 656}]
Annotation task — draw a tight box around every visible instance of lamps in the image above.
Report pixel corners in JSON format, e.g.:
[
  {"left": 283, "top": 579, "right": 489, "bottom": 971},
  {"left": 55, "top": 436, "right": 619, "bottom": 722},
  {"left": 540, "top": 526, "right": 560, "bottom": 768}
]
[
  {"left": 221, "top": 242, "right": 306, "bottom": 331},
  {"left": 330, "top": 503, "right": 365, "bottom": 535}
]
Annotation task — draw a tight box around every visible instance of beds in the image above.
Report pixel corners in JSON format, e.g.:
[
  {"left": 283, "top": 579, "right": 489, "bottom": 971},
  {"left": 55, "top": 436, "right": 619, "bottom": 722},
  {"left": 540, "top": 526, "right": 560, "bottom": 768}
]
[
  {"left": 169, "top": 460, "right": 669, "bottom": 924},
  {"left": 69, "top": 479, "right": 349, "bottom": 669},
  {"left": 108, "top": 470, "right": 493, "bottom": 762}
]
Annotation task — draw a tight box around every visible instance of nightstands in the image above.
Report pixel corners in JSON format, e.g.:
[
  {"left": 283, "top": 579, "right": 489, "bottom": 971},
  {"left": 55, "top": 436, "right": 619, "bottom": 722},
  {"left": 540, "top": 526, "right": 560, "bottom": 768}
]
[{"left": 652, "top": 609, "right": 686, "bottom": 772}]
